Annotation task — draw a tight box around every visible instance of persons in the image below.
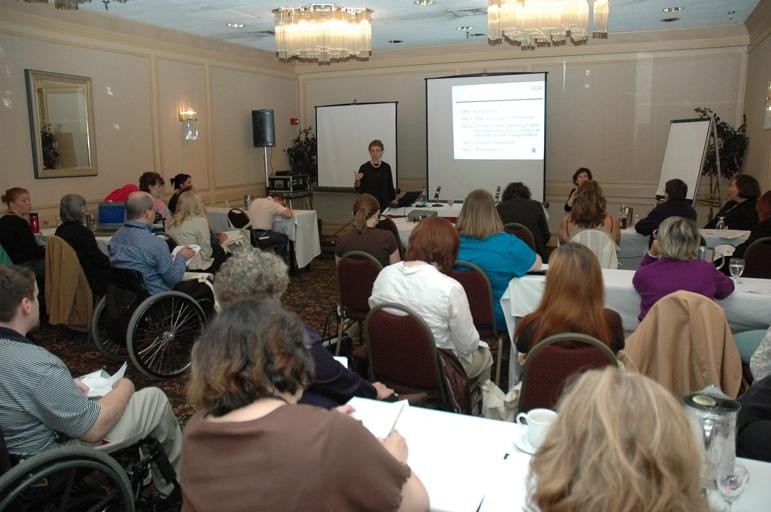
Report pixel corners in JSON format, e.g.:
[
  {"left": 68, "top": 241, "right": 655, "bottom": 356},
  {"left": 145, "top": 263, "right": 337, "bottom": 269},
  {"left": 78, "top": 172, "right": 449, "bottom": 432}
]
[
  {"left": 138, "top": 171, "right": 173, "bottom": 225},
  {"left": 247, "top": 194, "right": 293, "bottom": 262},
  {"left": 1, "top": 187, "right": 50, "bottom": 286},
  {"left": 352, "top": 139, "right": 396, "bottom": 213},
  {"left": 165, "top": 191, "right": 230, "bottom": 275},
  {"left": 54, "top": 194, "right": 116, "bottom": 295},
  {"left": 0, "top": 264, "right": 187, "bottom": 509},
  {"left": 106, "top": 191, "right": 217, "bottom": 329},
  {"left": 525, "top": 364, "right": 711, "bottom": 511},
  {"left": 175, "top": 297, "right": 432, "bottom": 510},
  {"left": 333, "top": 167, "right": 771, "bottom": 418},
  {"left": 168, "top": 174, "right": 193, "bottom": 217}
]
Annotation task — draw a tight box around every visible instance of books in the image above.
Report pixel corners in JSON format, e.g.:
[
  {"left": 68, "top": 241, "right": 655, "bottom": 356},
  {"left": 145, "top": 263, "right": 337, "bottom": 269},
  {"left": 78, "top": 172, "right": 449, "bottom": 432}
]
[{"left": 77, "top": 361, "right": 127, "bottom": 397}]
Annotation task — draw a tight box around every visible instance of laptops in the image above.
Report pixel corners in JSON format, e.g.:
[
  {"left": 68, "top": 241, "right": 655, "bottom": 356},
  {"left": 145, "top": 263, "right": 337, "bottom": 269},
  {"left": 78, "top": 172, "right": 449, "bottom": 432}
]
[
  {"left": 93, "top": 203, "right": 127, "bottom": 237},
  {"left": 389, "top": 191, "right": 420, "bottom": 207}
]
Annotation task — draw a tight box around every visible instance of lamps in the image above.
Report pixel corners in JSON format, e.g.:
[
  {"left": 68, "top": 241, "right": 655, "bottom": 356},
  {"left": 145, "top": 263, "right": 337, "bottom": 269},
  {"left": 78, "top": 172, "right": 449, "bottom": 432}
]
[
  {"left": 489, "top": 0, "right": 612, "bottom": 56},
  {"left": 271, "top": 5, "right": 376, "bottom": 64},
  {"left": 178, "top": 101, "right": 198, "bottom": 140}
]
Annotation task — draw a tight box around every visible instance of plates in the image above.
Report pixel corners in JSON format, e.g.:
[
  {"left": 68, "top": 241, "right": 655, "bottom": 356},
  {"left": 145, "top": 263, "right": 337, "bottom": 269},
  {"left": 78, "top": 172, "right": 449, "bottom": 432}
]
[{"left": 512, "top": 433, "right": 538, "bottom": 456}]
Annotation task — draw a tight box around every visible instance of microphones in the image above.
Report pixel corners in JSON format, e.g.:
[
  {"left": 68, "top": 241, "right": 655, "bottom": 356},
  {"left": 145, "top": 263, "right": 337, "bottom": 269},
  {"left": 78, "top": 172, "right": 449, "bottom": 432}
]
[
  {"left": 422, "top": 185, "right": 427, "bottom": 196},
  {"left": 435, "top": 185, "right": 441, "bottom": 198},
  {"left": 495, "top": 184, "right": 502, "bottom": 198}
]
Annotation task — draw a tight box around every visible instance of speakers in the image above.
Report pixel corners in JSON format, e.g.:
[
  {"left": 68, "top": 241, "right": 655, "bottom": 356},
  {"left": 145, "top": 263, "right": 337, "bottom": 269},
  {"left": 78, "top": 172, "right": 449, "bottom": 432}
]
[{"left": 252, "top": 109, "right": 274, "bottom": 148}]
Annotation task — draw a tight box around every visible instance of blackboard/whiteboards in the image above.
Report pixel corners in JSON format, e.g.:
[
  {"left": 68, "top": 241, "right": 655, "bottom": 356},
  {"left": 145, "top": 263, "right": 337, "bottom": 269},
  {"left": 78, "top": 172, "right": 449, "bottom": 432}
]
[{"left": 656, "top": 117, "right": 710, "bottom": 204}]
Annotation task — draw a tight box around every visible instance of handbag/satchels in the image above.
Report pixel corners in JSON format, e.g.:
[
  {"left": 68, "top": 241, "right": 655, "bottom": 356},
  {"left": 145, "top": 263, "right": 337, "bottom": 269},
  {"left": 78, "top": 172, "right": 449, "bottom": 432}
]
[{"left": 321, "top": 333, "right": 352, "bottom": 367}]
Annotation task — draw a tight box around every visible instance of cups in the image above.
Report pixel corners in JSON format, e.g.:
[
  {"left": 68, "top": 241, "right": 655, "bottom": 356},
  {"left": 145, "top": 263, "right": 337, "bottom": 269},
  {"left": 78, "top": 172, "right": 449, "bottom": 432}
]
[{"left": 515, "top": 407, "right": 558, "bottom": 449}]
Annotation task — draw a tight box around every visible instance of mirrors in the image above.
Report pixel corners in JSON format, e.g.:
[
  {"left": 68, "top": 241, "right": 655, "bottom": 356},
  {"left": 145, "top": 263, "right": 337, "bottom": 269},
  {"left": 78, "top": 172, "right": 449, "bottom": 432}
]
[{"left": 22, "top": 67, "right": 102, "bottom": 179}]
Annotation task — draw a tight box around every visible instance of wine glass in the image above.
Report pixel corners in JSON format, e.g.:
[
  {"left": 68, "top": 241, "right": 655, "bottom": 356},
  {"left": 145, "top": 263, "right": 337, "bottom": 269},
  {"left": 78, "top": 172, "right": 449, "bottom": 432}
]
[
  {"left": 728, "top": 257, "right": 746, "bottom": 283},
  {"left": 715, "top": 216, "right": 726, "bottom": 232},
  {"left": 715, "top": 461, "right": 749, "bottom": 512}
]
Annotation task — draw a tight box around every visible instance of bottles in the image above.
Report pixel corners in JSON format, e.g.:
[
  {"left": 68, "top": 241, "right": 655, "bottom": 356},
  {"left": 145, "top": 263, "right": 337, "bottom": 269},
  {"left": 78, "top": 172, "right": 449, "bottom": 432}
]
[
  {"left": 28, "top": 212, "right": 39, "bottom": 233},
  {"left": 634, "top": 214, "right": 640, "bottom": 224},
  {"left": 618, "top": 203, "right": 627, "bottom": 229}
]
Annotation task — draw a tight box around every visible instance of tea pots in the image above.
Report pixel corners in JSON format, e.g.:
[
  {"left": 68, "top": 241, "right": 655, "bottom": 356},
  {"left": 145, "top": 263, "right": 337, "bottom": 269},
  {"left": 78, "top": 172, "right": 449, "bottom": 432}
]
[
  {"left": 81, "top": 212, "right": 94, "bottom": 229},
  {"left": 696, "top": 246, "right": 725, "bottom": 270},
  {"left": 243, "top": 194, "right": 254, "bottom": 210},
  {"left": 681, "top": 384, "right": 742, "bottom": 490}
]
